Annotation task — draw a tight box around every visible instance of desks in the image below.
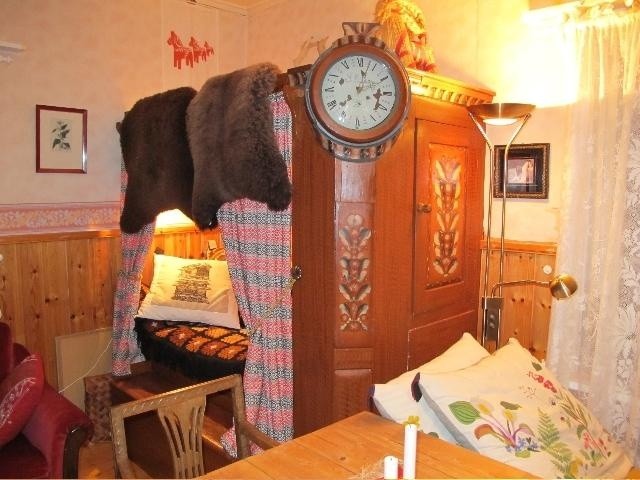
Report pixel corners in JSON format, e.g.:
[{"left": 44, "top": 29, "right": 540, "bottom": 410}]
[{"left": 182, "top": 404, "right": 543, "bottom": 478}]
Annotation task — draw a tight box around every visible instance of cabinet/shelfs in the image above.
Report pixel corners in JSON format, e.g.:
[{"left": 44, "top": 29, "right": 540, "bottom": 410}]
[{"left": 290, "top": 71, "right": 502, "bottom": 440}]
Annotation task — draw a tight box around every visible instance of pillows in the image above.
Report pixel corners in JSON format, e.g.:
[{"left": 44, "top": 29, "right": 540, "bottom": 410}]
[
  {"left": 1, "top": 352, "right": 44, "bottom": 443},
  {"left": 142, "top": 247, "right": 246, "bottom": 336},
  {"left": 370, "top": 332, "right": 494, "bottom": 447},
  {"left": 415, "top": 337, "right": 635, "bottom": 479}
]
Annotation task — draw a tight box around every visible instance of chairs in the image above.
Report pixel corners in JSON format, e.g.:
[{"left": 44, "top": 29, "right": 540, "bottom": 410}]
[
  {"left": 108, "top": 373, "right": 282, "bottom": 479},
  {"left": 3, "top": 322, "right": 96, "bottom": 480}
]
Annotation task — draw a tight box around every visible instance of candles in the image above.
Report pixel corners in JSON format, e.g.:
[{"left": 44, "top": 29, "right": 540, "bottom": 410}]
[
  {"left": 400, "top": 423, "right": 423, "bottom": 479},
  {"left": 382, "top": 452, "right": 399, "bottom": 480}
]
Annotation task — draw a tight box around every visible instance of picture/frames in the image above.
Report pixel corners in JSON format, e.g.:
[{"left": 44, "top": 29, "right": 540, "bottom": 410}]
[
  {"left": 491, "top": 139, "right": 550, "bottom": 201},
  {"left": 34, "top": 105, "right": 90, "bottom": 178}
]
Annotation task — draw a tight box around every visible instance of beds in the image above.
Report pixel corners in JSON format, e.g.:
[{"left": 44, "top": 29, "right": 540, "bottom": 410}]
[{"left": 114, "top": 72, "right": 295, "bottom": 403}]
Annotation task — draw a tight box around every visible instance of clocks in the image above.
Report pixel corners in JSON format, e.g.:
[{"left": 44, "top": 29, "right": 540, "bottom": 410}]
[{"left": 302, "top": 22, "right": 412, "bottom": 163}]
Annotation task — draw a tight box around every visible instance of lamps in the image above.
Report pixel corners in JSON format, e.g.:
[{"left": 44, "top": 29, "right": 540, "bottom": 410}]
[{"left": 467, "top": 103, "right": 577, "bottom": 355}]
